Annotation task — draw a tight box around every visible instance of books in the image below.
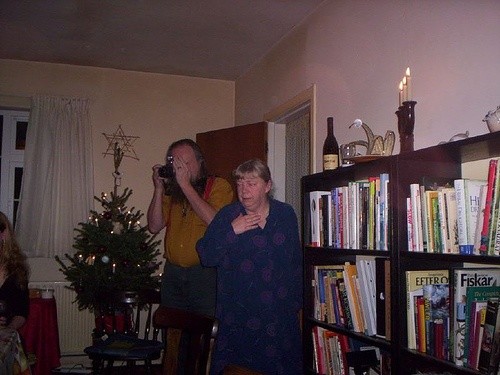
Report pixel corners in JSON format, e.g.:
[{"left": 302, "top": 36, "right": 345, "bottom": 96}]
[
  {"left": 406, "top": 158, "right": 500, "bottom": 256},
  {"left": 312, "top": 254, "right": 391, "bottom": 341},
  {"left": 311, "top": 325, "right": 391, "bottom": 375},
  {"left": 309, "top": 172, "right": 389, "bottom": 251},
  {"left": 405, "top": 262, "right": 500, "bottom": 375}
]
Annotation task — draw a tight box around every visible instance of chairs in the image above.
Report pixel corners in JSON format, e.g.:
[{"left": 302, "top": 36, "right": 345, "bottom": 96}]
[{"left": 82, "top": 277, "right": 171, "bottom": 375}]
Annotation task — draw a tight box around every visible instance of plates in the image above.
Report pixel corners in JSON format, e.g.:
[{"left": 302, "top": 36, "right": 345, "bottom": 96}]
[{"left": 342, "top": 154, "right": 389, "bottom": 163}]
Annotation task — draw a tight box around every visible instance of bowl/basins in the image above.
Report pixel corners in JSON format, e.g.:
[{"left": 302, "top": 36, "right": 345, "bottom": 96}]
[{"left": 42, "top": 288, "right": 54, "bottom": 298}]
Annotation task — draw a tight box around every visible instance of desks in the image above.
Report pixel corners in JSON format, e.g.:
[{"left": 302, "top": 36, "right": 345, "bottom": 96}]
[{"left": 15, "top": 288, "right": 58, "bottom": 373}]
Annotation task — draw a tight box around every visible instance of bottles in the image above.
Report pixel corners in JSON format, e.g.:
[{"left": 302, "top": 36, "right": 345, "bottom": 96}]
[{"left": 322, "top": 117, "right": 339, "bottom": 171}]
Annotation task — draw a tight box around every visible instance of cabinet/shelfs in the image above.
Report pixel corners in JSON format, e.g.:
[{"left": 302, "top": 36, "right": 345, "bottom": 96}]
[{"left": 302, "top": 129, "right": 500, "bottom": 374}]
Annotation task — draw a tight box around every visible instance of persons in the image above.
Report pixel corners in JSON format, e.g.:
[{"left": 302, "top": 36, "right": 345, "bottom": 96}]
[
  {"left": 195, "top": 159, "right": 306, "bottom": 375},
  {"left": 147, "top": 140, "right": 233, "bottom": 375},
  {"left": 0, "top": 211, "right": 33, "bottom": 375}
]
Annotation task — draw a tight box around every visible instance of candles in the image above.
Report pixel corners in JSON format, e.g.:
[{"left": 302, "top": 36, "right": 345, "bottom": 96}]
[{"left": 397, "top": 67, "right": 412, "bottom": 108}]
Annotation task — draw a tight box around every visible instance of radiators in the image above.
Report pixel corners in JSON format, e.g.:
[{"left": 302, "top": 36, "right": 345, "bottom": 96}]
[{"left": 28, "top": 280, "right": 99, "bottom": 358}]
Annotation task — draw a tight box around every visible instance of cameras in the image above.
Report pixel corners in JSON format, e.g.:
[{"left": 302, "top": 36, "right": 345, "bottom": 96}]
[{"left": 158, "top": 156, "right": 176, "bottom": 178}]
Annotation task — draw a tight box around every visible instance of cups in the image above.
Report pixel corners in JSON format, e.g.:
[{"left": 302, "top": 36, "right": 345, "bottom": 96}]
[{"left": 340, "top": 144, "right": 356, "bottom": 167}]
